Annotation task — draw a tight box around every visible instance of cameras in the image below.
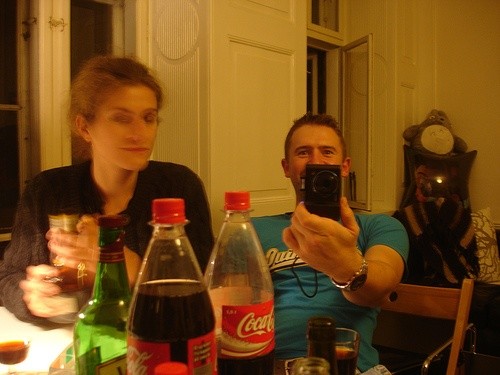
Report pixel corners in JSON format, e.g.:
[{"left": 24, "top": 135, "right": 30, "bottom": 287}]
[{"left": 306, "top": 164, "right": 342, "bottom": 222}]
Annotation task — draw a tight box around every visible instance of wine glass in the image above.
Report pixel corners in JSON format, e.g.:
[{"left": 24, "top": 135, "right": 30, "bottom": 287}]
[{"left": 0, "top": 331, "right": 31, "bottom": 375}]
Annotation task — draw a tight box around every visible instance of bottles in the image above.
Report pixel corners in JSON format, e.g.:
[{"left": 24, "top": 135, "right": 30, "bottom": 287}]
[
  {"left": 126, "top": 198, "right": 217, "bottom": 375},
  {"left": 335, "top": 326, "right": 359, "bottom": 375},
  {"left": 293, "top": 357, "right": 330, "bottom": 375},
  {"left": 305, "top": 316, "right": 338, "bottom": 375},
  {"left": 202, "top": 185, "right": 276, "bottom": 375},
  {"left": 72, "top": 216, "right": 133, "bottom": 375}
]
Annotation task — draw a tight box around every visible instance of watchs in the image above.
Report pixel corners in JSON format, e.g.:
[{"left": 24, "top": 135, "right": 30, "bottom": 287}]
[{"left": 330, "top": 248, "right": 369, "bottom": 292}]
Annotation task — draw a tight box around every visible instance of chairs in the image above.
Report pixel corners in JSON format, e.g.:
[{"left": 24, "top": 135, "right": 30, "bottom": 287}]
[{"left": 383, "top": 278, "right": 474, "bottom": 375}]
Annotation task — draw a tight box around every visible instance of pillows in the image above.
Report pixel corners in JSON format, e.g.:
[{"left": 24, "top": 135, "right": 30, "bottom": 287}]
[{"left": 472, "top": 207, "right": 500, "bottom": 282}]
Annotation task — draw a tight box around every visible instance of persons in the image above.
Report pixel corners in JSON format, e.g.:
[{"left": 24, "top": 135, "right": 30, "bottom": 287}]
[
  {"left": 0, "top": 55, "right": 216, "bottom": 331},
  {"left": 216, "top": 112, "right": 410, "bottom": 375}
]
